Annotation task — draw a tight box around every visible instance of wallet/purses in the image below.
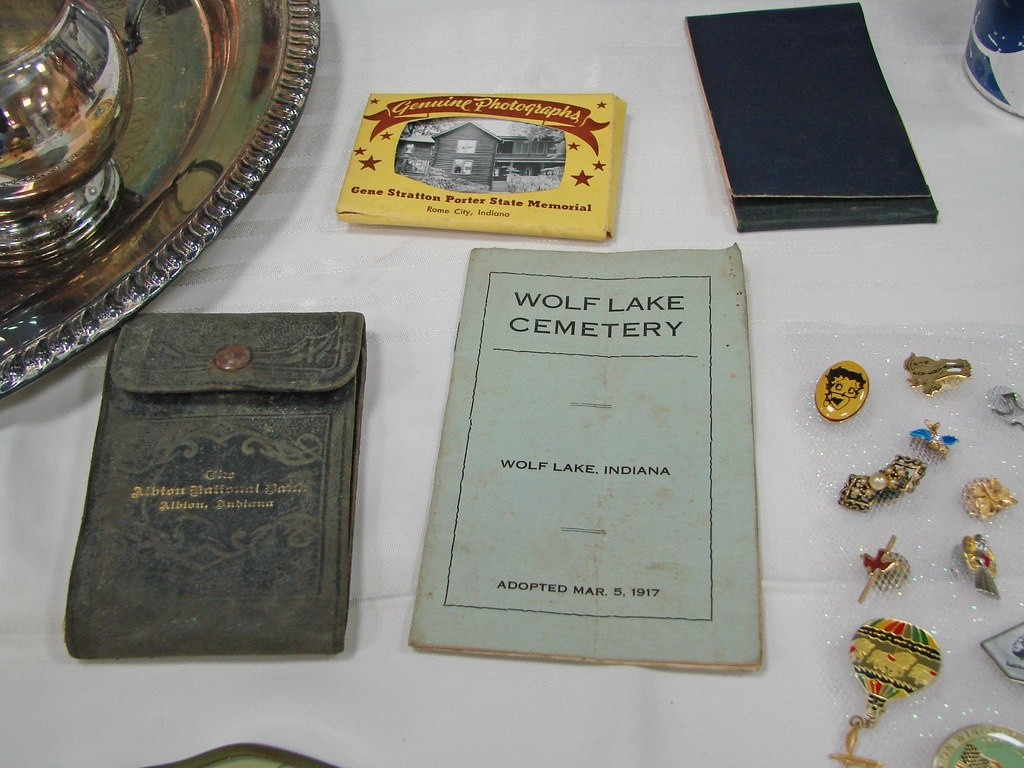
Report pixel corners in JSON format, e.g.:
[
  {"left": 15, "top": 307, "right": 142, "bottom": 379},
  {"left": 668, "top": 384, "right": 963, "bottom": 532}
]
[{"left": 65, "top": 311, "right": 365, "bottom": 658}]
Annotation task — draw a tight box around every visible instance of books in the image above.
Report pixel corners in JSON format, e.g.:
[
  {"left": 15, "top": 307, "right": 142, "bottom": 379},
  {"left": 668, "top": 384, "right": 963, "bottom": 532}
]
[
  {"left": 684, "top": 1, "right": 938, "bottom": 235},
  {"left": 408, "top": 239, "right": 765, "bottom": 675}
]
